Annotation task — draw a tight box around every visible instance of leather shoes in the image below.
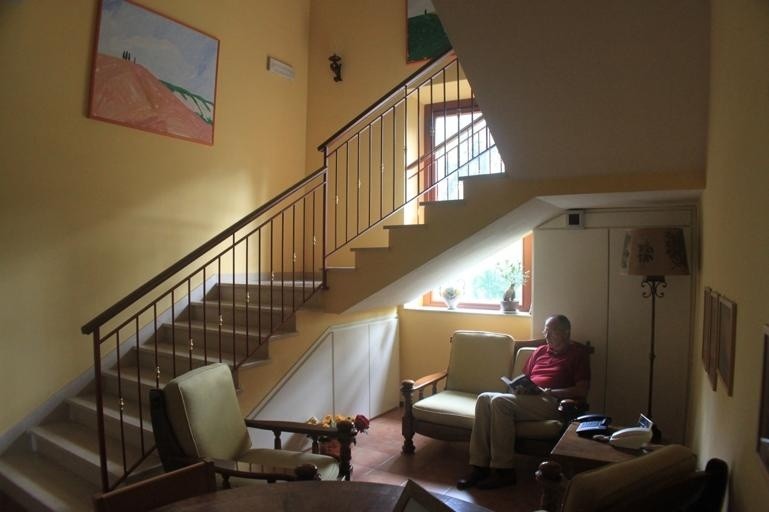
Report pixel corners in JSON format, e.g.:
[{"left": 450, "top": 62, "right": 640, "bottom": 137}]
[{"left": 457, "top": 469, "right": 516, "bottom": 490}]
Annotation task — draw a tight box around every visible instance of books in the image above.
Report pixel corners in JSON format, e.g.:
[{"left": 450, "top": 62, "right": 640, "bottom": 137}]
[{"left": 499, "top": 374, "right": 544, "bottom": 395}]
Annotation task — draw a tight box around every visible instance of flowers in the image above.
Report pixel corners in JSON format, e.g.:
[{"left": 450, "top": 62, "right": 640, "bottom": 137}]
[{"left": 304, "top": 413, "right": 370, "bottom": 446}]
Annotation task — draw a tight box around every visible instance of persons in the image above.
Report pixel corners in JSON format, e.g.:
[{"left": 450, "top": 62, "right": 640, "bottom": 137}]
[{"left": 456, "top": 313, "right": 592, "bottom": 491}]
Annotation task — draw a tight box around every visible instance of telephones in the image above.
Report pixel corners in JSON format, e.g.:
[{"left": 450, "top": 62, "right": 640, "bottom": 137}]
[
  {"left": 576, "top": 413, "right": 613, "bottom": 435},
  {"left": 593, "top": 427, "right": 652, "bottom": 448}
]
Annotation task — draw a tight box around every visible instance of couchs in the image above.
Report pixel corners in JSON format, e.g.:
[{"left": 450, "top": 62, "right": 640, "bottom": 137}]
[{"left": 400, "top": 329, "right": 595, "bottom": 455}]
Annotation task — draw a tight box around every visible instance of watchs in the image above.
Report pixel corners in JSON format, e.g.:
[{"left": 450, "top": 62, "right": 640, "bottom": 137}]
[{"left": 544, "top": 387, "right": 552, "bottom": 396}]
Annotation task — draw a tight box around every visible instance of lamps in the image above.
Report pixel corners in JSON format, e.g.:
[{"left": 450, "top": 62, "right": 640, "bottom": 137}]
[{"left": 617, "top": 226, "right": 691, "bottom": 442}]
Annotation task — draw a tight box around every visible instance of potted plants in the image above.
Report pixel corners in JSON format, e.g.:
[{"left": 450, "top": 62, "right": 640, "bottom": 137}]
[
  {"left": 440, "top": 286, "right": 463, "bottom": 310},
  {"left": 495, "top": 257, "right": 531, "bottom": 311}
]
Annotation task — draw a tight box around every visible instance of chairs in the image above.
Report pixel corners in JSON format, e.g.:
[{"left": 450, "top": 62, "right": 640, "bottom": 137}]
[
  {"left": 149, "top": 362, "right": 353, "bottom": 490},
  {"left": 532, "top": 457, "right": 728, "bottom": 512}
]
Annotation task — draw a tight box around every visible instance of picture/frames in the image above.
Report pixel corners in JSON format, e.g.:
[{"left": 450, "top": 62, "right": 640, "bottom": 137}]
[
  {"left": 700, "top": 285, "right": 738, "bottom": 398},
  {"left": 405, "top": 1, "right": 452, "bottom": 66},
  {"left": 85, "top": 0, "right": 220, "bottom": 146}
]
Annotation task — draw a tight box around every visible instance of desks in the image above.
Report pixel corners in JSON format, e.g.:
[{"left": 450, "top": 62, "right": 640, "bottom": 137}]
[{"left": 549, "top": 421, "right": 684, "bottom": 473}]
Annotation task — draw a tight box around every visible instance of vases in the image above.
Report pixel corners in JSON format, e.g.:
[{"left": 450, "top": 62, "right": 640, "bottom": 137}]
[{"left": 317, "top": 439, "right": 352, "bottom": 473}]
